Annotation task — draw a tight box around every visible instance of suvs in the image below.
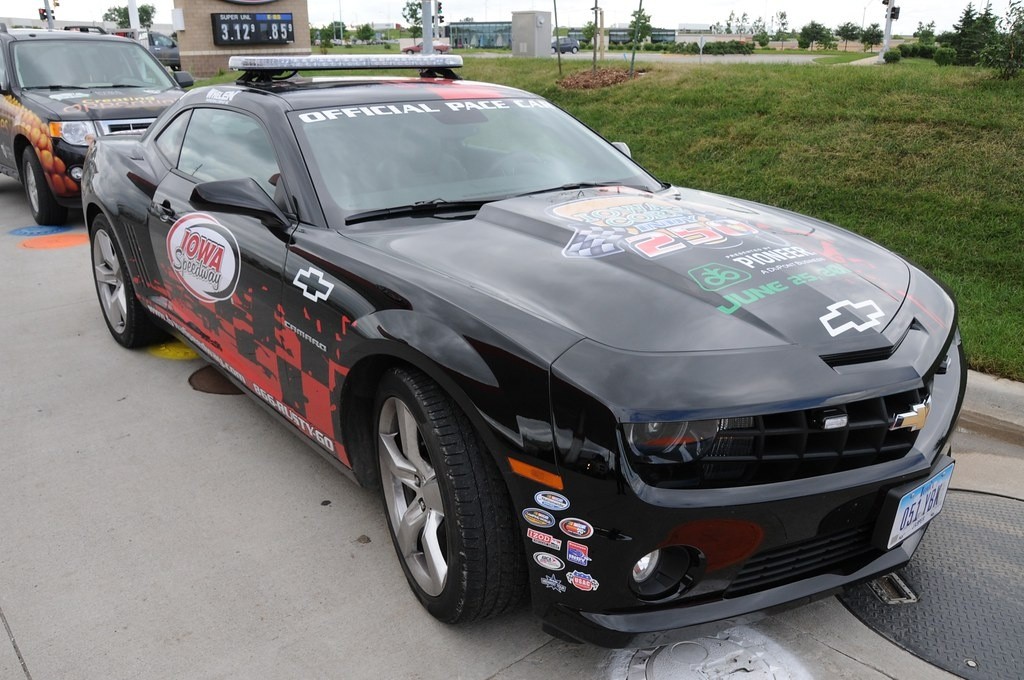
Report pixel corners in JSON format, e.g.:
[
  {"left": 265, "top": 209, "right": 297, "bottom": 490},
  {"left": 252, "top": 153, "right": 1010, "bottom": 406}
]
[{"left": 0, "top": 24, "right": 195, "bottom": 227}]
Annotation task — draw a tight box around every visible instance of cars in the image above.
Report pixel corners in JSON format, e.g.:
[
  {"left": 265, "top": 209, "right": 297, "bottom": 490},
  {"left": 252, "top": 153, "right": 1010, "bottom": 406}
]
[
  {"left": 551, "top": 36, "right": 581, "bottom": 55},
  {"left": 402, "top": 41, "right": 449, "bottom": 55},
  {"left": 109, "top": 29, "right": 181, "bottom": 71},
  {"left": 313, "top": 36, "right": 399, "bottom": 48},
  {"left": 82, "top": 52, "right": 971, "bottom": 652}
]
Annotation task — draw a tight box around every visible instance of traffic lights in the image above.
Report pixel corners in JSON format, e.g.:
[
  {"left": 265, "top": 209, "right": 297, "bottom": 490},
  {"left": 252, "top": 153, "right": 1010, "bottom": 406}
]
[
  {"left": 39, "top": 9, "right": 47, "bottom": 20},
  {"left": 438, "top": 2, "right": 443, "bottom": 14},
  {"left": 440, "top": 15, "right": 444, "bottom": 23},
  {"left": 882, "top": 0, "right": 888, "bottom": 5},
  {"left": 891, "top": 7, "right": 900, "bottom": 19}
]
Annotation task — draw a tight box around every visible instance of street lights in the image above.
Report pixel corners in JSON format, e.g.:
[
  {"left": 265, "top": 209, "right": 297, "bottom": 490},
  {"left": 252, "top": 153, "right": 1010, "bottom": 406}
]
[{"left": 781, "top": 23, "right": 785, "bottom": 50}]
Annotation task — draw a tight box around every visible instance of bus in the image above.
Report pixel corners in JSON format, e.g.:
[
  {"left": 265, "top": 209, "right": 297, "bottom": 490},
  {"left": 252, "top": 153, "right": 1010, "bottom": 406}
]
[{"left": 449, "top": 22, "right": 512, "bottom": 50}]
